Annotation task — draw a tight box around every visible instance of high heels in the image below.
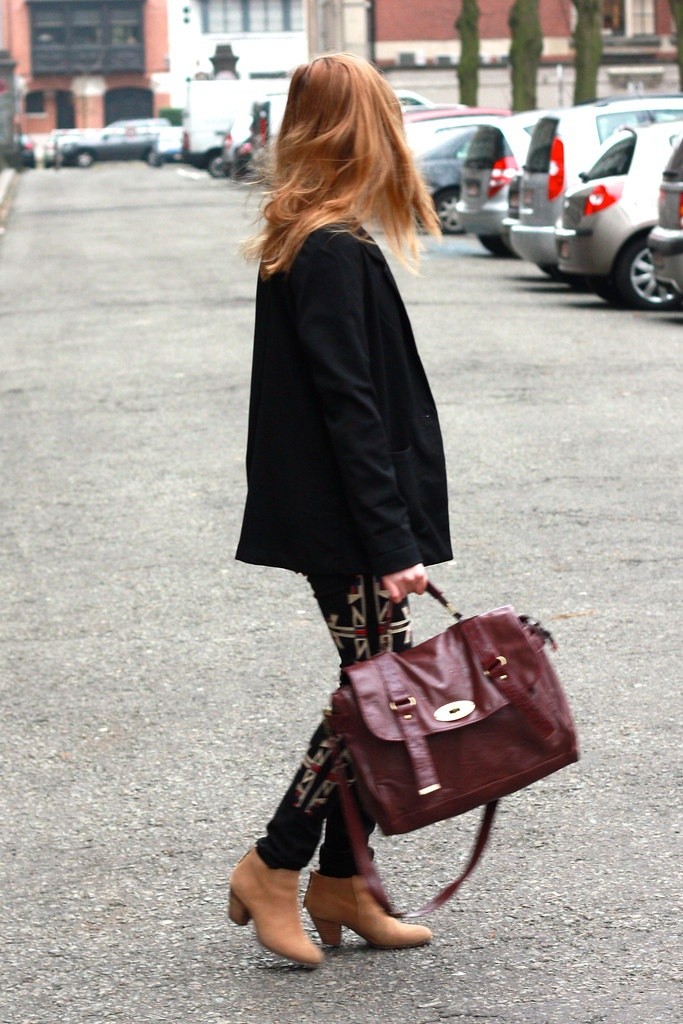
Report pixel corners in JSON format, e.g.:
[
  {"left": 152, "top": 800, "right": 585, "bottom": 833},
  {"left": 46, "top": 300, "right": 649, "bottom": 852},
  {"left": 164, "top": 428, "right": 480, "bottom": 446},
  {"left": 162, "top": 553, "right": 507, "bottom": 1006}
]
[
  {"left": 303, "top": 870, "right": 433, "bottom": 949},
  {"left": 227, "top": 847, "right": 323, "bottom": 965}
]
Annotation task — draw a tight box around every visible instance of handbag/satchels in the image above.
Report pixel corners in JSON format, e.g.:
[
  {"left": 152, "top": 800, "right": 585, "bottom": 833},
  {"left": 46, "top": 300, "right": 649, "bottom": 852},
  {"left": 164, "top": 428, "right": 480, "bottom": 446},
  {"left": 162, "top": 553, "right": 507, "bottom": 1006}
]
[{"left": 332, "top": 582, "right": 580, "bottom": 836}]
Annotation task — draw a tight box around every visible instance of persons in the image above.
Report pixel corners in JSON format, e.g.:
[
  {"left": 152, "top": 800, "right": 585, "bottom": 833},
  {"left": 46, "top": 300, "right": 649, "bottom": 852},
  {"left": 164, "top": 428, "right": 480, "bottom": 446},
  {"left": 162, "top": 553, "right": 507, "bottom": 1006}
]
[{"left": 226, "top": 51, "right": 456, "bottom": 967}]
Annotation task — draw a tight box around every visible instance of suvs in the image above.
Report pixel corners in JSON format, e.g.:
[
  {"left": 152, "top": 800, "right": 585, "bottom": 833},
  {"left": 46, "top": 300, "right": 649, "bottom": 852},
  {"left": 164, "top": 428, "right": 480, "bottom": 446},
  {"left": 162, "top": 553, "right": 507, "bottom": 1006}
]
[{"left": 510, "top": 95, "right": 683, "bottom": 293}]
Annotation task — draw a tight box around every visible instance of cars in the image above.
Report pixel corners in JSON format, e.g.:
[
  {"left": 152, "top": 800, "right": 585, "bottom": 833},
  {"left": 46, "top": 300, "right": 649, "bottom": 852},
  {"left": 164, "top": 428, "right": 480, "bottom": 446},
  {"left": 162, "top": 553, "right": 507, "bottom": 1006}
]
[
  {"left": 647, "top": 132, "right": 683, "bottom": 299},
  {"left": 553, "top": 123, "right": 683, "bottom": 310},
  {"left": 13, "top": 75, "right": 509, "bottom": 182},
  {"left": 410, "top": 129, "right": 478, "bottom": 237},
  {"left": 450, "top": 110, "right": 568, "bottom": 260}
]
